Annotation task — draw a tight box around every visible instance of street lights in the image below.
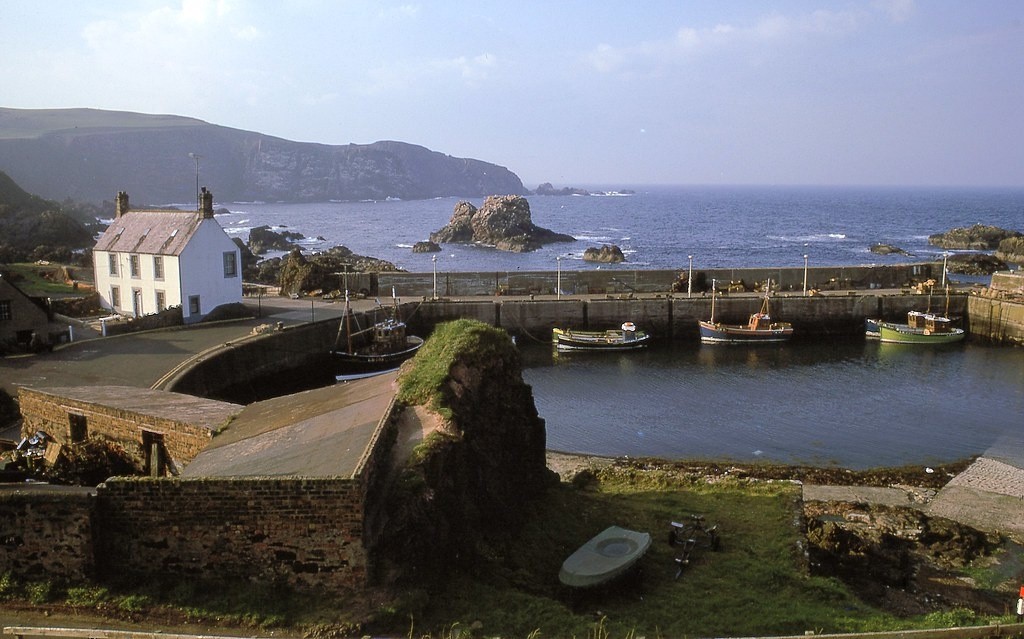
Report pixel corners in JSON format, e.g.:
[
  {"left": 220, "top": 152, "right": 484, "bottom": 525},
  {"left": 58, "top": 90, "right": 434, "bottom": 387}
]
[
  {"left": 188, "top": 152, "right": 201, "bottom": 212},
  {"left": 431, "top": 255, "right": 439, "bottom": 300},
  {"left": 803, "top": 253, "right": 809, "bottom": 297},
  {"left": 942, "top": 252, "right": 951, "bottom": 287},
  {"left": 557, "top": 257, "right": 563, "bottom": 302},
  {"left": 687, "top": 255, "right": 692, "bottom": 299}
]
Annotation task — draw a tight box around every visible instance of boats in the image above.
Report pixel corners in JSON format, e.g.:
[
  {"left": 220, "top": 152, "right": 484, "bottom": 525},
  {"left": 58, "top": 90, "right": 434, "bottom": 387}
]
[
  {"left": 879, "top": 285, "right": 965, "bottom": 345},
  {"left": 325, "top": 264, "right": 426, "bottom": 369},
  {"left": 550, "top": 320, "right": 651, "bottom": 357},
  {"left": 557, "top": 526, "right": 652, "bottom": 591},
  {"left": 699, "top": 279, "right": 794, "bottom": 345},
  {"left": 862, "top": 294, "right": 936, "bottom": 341}
]
